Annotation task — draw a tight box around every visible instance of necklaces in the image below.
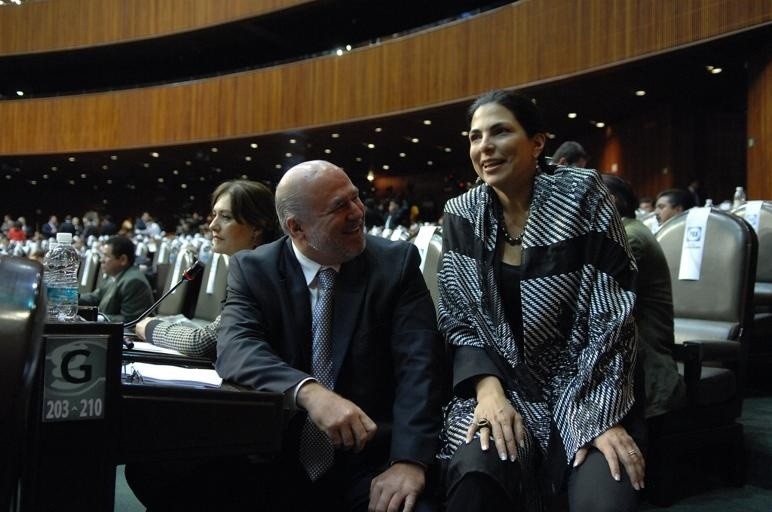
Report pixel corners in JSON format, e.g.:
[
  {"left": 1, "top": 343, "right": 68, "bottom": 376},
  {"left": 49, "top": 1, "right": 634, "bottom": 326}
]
[{"left": 502, "top": 225, "right": 523, "bottom": 246}]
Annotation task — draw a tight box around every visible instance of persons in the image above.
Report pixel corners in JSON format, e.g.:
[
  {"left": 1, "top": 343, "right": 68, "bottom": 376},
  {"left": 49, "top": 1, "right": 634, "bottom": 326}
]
[
  {"left": 436, "top": 89, "right": 645, "bottom": 512},
  {"left": 600, "top": 173, "right": 686, "bottom": 421},
  {"left": 553, "top": 141, "right": 588, "bottom": 169},
  {"left": 216, "top": 160, "right": 437, "bottom": 512},
  {"left": 125, "top": 179, "right": 285, "bottom": 511},
  {"left": 361, "top": 197, "right": 412, "bottom": 230},
  {"left": 637, "top": 189, "right": 690, "bottom": 230},
  {"left": 1, "top": 212, "right": 212, "bottom": 265},
  {"left": 76, "top": 236, "right": 153, "bottom": 324}
]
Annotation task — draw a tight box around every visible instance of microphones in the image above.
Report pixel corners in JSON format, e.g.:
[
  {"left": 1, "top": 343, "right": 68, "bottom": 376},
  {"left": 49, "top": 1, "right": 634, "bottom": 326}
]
[{"left": 98, "top": 260, "right": 205, "bottom": 329}]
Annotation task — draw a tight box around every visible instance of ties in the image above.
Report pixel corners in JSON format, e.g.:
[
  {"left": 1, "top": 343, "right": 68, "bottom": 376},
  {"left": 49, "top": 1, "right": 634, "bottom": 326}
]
[{"left": 297, "top": 267, "right": 338, "bottom": 484}]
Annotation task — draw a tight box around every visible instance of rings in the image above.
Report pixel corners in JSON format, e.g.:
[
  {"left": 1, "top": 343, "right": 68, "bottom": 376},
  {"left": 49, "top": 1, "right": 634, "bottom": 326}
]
[
  {"left": 627, "top": 450, "right": 637, "bottom": 455},
  {"left": 476, "top": 419, "right": 490, "bottom": 426}
]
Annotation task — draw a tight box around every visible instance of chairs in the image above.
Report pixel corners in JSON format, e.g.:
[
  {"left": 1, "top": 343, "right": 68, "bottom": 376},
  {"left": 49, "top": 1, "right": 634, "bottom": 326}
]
[{"left": 1, "top": 199, "right": 772, "bottom": 512}]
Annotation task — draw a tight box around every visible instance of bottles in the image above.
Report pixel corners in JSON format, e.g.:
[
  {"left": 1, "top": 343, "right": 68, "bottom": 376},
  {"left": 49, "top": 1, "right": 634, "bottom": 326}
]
[
  {"left": 44, "top": 232, "right": 78, "bottom": 319},
  {"left": 703, "top": 187, "right": 746, "bottom": 210}
]
